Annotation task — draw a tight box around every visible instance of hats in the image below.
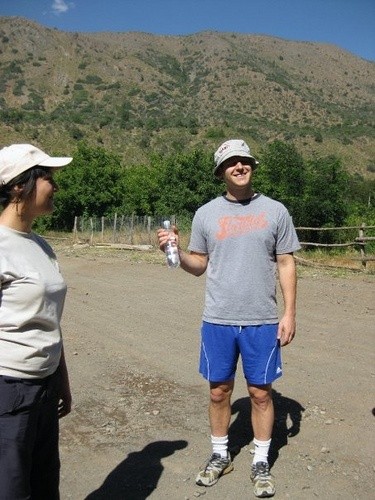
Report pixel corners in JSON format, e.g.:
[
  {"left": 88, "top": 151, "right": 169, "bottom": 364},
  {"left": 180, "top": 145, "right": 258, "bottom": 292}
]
[
  {"left": 0, "top": 144, "right": 73, "bottom": 186},
  {"left": 213, "top": 139, "right": 259, "bottom": 179}
]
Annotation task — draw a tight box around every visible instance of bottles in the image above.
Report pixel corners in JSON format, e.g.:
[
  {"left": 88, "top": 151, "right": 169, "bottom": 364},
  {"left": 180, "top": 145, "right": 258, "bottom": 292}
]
[{"left": 163, "top": 220, "right": 181, "bottom": 270}]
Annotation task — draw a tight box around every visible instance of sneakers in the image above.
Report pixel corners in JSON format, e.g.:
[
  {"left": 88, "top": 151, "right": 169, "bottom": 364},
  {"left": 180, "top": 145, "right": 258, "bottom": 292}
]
[
  {"left": 196, "top": 451, "right": 235, "bottom": 487},
  {"left": 249, "top": 458, "right": 276, "bottom": 497}
]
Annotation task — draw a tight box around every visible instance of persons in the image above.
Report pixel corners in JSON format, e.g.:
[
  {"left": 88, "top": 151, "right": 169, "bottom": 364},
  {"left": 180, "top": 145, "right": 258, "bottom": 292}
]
[
  {"left": 0, "top": 143, "right": 74, "bottom": 500},
  {"left": 157, "top": 139, "right": 302, "bottom": 498}
]
